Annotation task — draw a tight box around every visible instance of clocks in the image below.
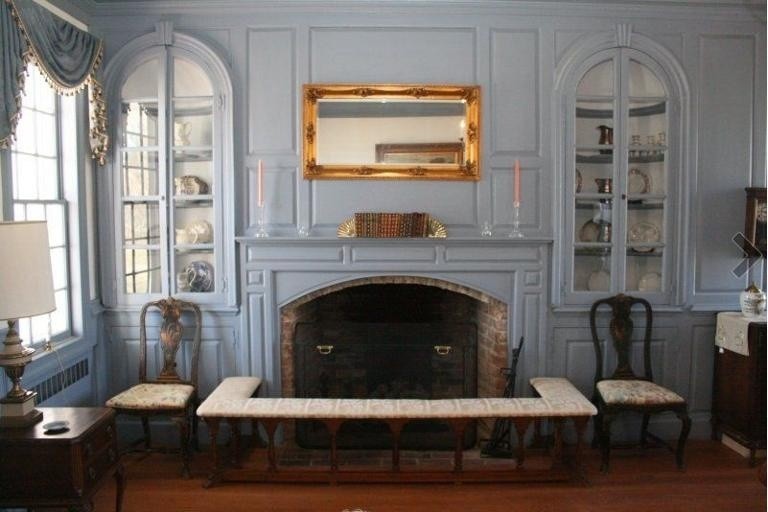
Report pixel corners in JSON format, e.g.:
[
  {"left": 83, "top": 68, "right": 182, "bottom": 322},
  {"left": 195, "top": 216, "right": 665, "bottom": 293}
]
[{"left": 745, "top": 188, "right": 767, "bottom": 257}]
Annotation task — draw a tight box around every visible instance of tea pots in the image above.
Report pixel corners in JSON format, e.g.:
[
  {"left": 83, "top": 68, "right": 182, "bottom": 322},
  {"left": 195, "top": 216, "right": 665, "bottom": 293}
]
[
  {"left": 593, "top": 124, "right": 613, "bottom": 158},
  {"left": 173, "top": 120, "right": 193, "bottom": 147}
]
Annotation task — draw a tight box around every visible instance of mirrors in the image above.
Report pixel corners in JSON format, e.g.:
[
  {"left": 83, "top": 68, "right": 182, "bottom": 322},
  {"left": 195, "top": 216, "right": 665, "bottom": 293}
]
[{"left": 302, "top": 84, "right": 480, "bottom": 182}]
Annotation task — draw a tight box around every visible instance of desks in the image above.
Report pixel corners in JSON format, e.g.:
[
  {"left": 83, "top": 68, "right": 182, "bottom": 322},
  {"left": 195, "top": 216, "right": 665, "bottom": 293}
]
[{"left": 1, "top": 407, "right": 124, "bottom": 511}]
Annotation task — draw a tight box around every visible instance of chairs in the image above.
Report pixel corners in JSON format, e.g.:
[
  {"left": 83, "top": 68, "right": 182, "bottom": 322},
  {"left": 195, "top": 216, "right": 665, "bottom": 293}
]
[
  {"left": 106, "top": 298, "right": 202, "bottom": 480},
  {"left": 591, "top": 294, "right": 692, "bottom": 476}
]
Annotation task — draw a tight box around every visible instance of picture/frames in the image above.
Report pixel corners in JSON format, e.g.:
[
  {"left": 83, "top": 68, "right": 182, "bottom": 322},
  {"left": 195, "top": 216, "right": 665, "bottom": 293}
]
[{"left": 375, "top": 143, "right": 463, "bottom": 164}]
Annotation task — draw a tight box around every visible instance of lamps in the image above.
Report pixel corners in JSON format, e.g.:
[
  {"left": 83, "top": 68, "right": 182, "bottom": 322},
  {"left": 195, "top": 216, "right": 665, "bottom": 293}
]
[{"left": 0, "top": 220, "right": 56, "bottom": 429}]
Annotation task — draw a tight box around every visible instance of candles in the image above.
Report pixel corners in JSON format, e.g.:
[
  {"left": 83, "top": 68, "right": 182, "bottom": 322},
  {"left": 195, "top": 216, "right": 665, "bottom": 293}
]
[
  {"left": 258, "top": 160, "right": 263, "bottom": 207},
  {"left": 514, "top": 159, "right": 520, "bottom": 201}
]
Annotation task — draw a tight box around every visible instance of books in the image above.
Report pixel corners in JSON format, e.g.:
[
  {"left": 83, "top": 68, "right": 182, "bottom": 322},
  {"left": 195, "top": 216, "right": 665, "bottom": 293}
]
[{"left": 354, "top": 211, "right": 429, "bottom": 238}]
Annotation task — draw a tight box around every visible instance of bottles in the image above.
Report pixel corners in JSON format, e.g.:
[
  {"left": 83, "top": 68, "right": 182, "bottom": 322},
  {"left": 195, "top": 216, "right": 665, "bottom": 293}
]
[
  {"left": 480, "top": 220, "right": 494, "bottom": 239},
  {"left": 296, "top": 223, "right": 309, "bottom": 240},
  {"left": 627, "top": 130, "right": 667, "bottom": 164}
]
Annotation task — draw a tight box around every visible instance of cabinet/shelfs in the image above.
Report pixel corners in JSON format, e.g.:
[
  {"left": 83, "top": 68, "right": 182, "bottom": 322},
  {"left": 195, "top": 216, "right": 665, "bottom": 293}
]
[
  {"left": 711, "top": 318, "right": 767, "bottom": 465},
  {"left": 109, "top": 45, "right": 224, "bottom": 305},
  {"left": 562, "top": 46, "right": 681, "bottom": 306}
]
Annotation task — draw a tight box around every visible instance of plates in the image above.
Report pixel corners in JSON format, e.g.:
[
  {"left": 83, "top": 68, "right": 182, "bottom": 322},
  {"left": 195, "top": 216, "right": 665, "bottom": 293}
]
[
  {"left": 172, "top": 175, "right": 213, "bottom": 296},
  {"left": 575, "top": 168, "right": 663, "bottom": 292},
  {"left": 41, "top": 419, "right": 73, "bottom": 434}
]
